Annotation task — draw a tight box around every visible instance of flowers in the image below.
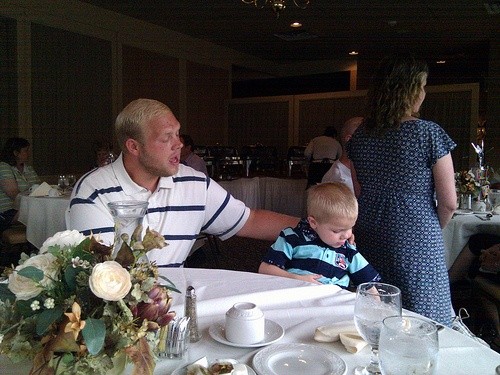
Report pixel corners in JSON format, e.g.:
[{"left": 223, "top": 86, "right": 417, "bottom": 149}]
[
  {"left": 453, "top": 170, "right": 484, "bottom": 196},
  {"left": 0, "top": 219, "right": 189, "bottom": 375}
]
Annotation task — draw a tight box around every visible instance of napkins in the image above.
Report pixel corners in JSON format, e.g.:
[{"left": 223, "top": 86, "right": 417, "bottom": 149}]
[
  {"left": 313, "top": 319, "right": 367, "bottom": 354},
  {"left": 28, "top": 181, "right": 61, "bottom": 197}
]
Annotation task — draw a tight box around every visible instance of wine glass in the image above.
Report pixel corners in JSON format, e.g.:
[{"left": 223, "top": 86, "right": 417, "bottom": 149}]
[
  {"left": 58, "top": 178, "right": 67, "bottom": 194},
  {"left": 488, "top": 193, "right": 500, "bottom": 215},
  {"left": 354, "top": 283, "right": 402, "bottom": 375},
  {"left": 66, "top": 174, "right": 76, "bottom": 191}
]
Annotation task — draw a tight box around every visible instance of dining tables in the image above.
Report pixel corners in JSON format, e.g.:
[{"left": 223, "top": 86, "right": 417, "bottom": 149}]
[
  {"left": 13, "top": 184, "right": 73, "bottom": 250},
  {"left": 0, "top": 267, "right": 500, "bottom": 375},
  {"left": 437, "top": 198, "right": 500, "bottom": 273}
]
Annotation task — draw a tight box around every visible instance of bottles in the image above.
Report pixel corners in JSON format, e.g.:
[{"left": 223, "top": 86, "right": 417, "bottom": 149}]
[
  {"left": 185, "top": 286, "right": 201, "bottom": 343},
  {"left": 466, "top": 194, "right": 471, "bottom": 210},
  {"left": 459, "top": 194, "right": 464, "bottom": 209}
]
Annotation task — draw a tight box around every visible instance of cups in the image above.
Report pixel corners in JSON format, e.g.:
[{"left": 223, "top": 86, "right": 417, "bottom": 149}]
[
  {"left": 473, "top": 201, "right": 485, "bottom": 212},
  {"left": 29, "top": 184, "right": 39, "bottom": 193},
  {"left": 49, "top": 189, "right": 58, "bottom": 197},
  {"left": 378, "top": 316, "right": 439, "bottom": 375},
  {"left": 166, "top": 330, "right": 190, "bottom": 357},
  {"left": 225, "top": 302, "right": 264, "bottom": 343}
]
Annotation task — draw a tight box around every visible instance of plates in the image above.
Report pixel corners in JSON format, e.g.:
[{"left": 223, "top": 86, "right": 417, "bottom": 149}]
[
  {"left": 472, "top": 211, "right": 490, "bottom": 213},
  {"left": 208, "top": 319, "right": 283, "bottom": 347},
  {"left": 252, "top": 344, "right": 347, "bottom": 375},
  {"left": 172, "top": 360, "right": 256, "bottom": 375},
  {"left": 45, "top": 195, "right": 63, "bottom": 197},
  {"left": 455, "top": 210, "right": 472, "bottom": 214}
]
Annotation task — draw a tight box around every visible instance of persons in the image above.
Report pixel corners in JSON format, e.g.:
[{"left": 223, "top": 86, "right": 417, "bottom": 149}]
[
  {"left": 447, "top": 233, "right": 500, "bottom": 347},
  {"left": 258, "top": 182, "right": 381, "bottom": 300},
  {"left": 62, "top": 99, "right": 356, "bottom": 269},
  {"left": 304, "top": 125, "right": 342, "bottom": 192},
  {"left": 322, "top": 116, "right": 368, "bottom": 198},
  {"left": 178, "top": 132, "right": 209, "bottom": 176},
  {"left": 343, "top": 57, "right": 457, "bottom": 333},
  {"left": 0, "top": 137, "right": 42, "bottom": 224},
  {"left": 92, "top": 145, "right": 111, "bottom": 166}
]
